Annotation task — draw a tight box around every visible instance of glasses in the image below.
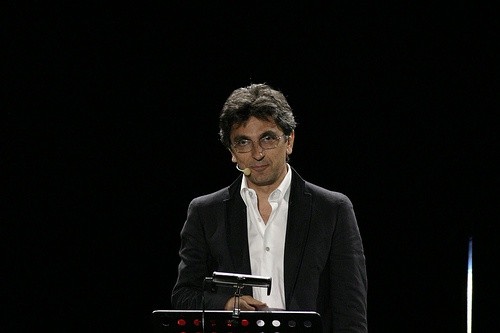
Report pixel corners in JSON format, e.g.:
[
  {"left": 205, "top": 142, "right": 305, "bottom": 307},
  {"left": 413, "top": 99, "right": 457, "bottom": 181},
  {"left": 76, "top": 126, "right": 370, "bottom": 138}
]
[{"left": 230, "top": 134, "right": 287, "bottom": 153}]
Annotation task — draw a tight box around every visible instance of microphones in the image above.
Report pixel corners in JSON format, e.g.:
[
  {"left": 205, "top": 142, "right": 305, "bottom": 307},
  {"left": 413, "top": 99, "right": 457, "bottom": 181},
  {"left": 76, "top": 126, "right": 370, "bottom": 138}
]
[{"left": 236, "top": 163, "right": 251, "bottom": 176}]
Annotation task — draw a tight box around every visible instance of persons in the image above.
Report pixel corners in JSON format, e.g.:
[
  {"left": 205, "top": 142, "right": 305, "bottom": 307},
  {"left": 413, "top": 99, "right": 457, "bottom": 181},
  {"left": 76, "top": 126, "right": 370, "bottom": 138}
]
[{"left": 170, "top": 83, "right": 368, "bottom": 333}]
